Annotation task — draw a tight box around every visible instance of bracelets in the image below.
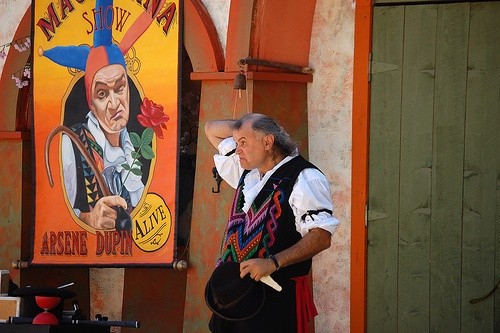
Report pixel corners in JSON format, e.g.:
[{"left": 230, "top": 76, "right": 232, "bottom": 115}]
[{"left": 268, "top": 255, "right": 280, "bottom": 271}]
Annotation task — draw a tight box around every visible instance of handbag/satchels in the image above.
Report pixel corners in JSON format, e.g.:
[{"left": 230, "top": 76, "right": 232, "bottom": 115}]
[{"left": 204, "top": 261, "right": 270, "bottom": 321}]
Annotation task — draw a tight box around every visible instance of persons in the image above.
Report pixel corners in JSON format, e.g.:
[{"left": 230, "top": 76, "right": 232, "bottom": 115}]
[{"left": 205, "top": 114, "right": 340, "bottom": 333}]
